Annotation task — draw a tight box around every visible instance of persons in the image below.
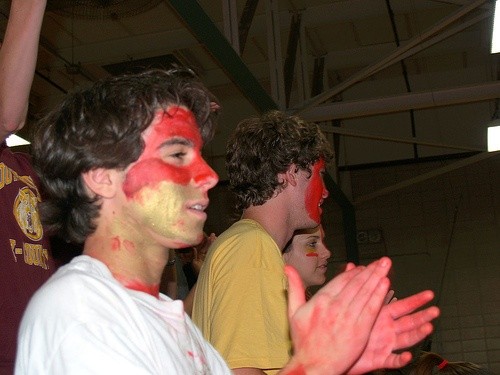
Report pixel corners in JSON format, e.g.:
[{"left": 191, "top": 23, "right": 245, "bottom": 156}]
[
  {"left": 280, "top": 225, "right": 396, "bottom": 305},
  {"left": 192, "top": 111, "right": 335, "bottom": 375},
  {"left": 162, "top": 232, "right": 218, "bottom": 316},
  {"left": 1, "top": 0, "right": 59, "bottom": 375},
  {"left": 14, "top": 72, "right": 441, "bottom": 375}
]
